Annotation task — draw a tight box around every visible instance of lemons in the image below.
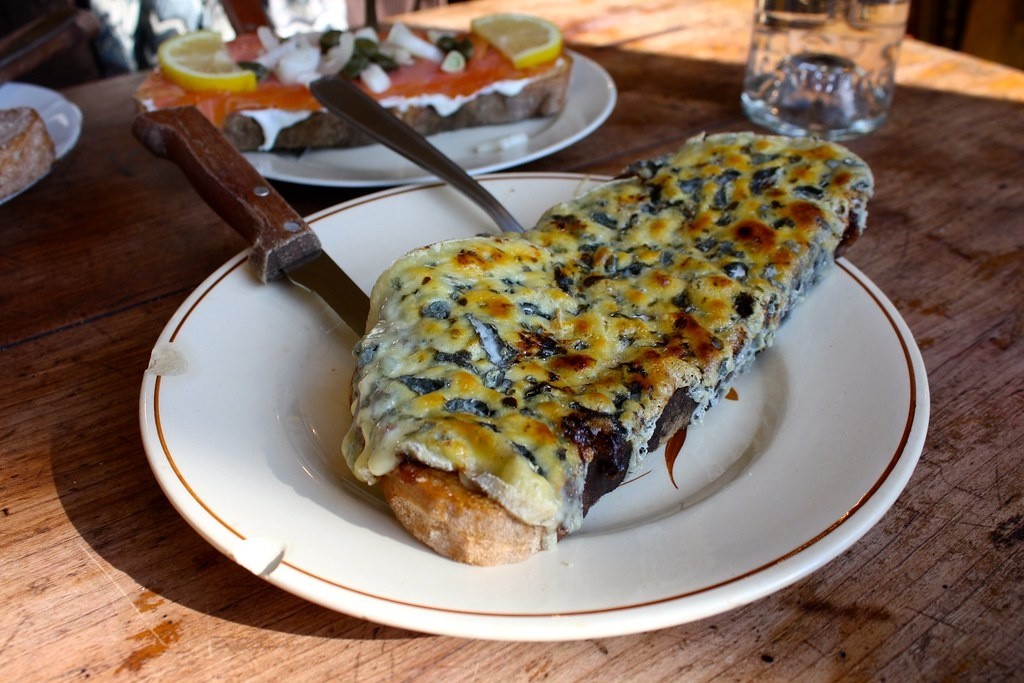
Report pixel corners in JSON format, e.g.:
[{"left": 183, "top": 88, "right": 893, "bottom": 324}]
[
  {"left": 157, "top": 29, "right": 258, "bottom": 92},
  {"left": 470, "top": 13, "right": 562, "bottom": 69}
]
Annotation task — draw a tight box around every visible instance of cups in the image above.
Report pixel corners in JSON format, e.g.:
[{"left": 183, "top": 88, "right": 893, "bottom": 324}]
[{"left": 737, "top": 1, "right": 913, "bottom": 143}]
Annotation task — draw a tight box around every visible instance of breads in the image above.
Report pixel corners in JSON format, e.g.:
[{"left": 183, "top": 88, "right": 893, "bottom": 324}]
[
  {"left": 0, "top": 106, "right": 55, "bottom": 200},
  {"left": 132, "top": 55, "right": 572, "bottom": 151}
]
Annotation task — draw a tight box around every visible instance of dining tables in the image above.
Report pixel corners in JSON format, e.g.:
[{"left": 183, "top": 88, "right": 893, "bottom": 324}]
[{"left": 0, "top": 0, "right": 1024, "bottom": 683}]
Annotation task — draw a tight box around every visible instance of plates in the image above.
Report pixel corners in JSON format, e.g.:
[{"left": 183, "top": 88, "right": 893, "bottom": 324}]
[
  {"left": 237, "top": 47, "right": 621, "bottom": 190},
  {"left": 138, "top": 172, "right": 933, "bottom": 646},
  {"left": 0, "top": 79, "right": 85, "bottom": 207}
]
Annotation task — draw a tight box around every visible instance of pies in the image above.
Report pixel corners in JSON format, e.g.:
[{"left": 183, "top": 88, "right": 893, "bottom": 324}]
[{"left": 341, "top": 132, "right": 875, "bottom": 566}]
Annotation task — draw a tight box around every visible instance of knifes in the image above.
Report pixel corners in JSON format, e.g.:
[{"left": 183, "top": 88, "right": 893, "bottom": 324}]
[{"left": 130, "top": 105, "right": 370, "bottom": 340}]
